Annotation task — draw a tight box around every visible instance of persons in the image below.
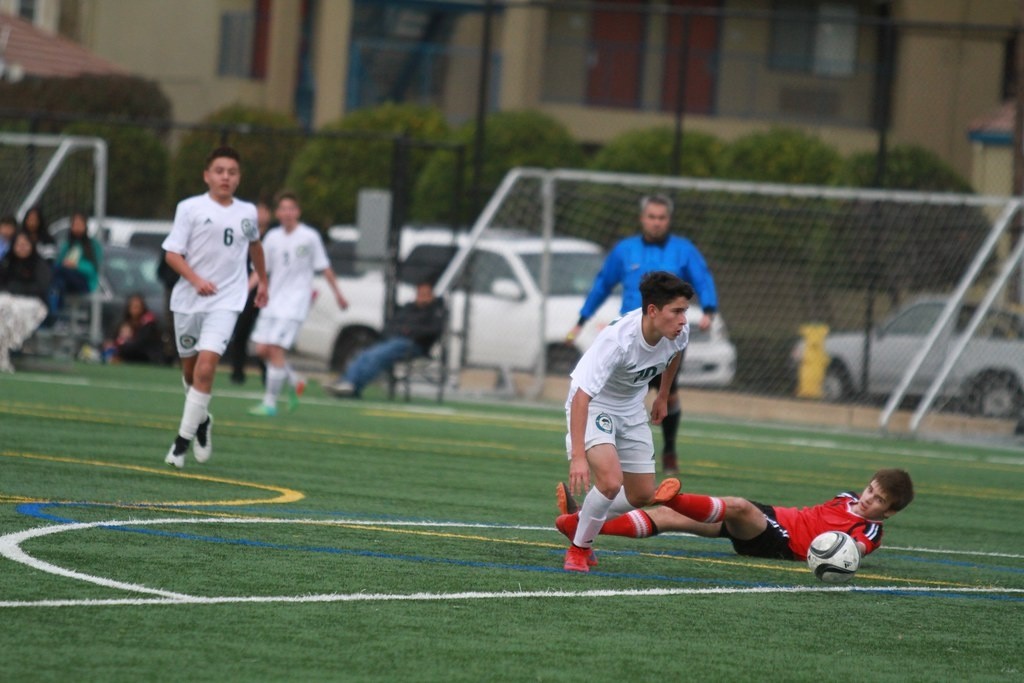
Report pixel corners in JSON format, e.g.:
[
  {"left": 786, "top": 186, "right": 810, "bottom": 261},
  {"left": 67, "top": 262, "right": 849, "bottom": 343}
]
[
  {"left": 557, "top": 469, "right": 914, "bottom": 561},
  {"left": 0, "top": 208, "right": 104, "bottom": 327},
  {"left": 568, "top": 190, "right": 721, "bottom": 476},
  {"left": 555, "top": 271, "right": 695, "bottom": 573},
  {"left": 162, "top": 146, "right": 269, "bottom": 468},
  {"left": 231, "top": 195, "right": 348, "bottom": 416},
  {"left": 320, "top": 282, "right": 451, "bottom": 398},
  {"left": 102, "top": 292, "right": 161, "bottom": 366}
]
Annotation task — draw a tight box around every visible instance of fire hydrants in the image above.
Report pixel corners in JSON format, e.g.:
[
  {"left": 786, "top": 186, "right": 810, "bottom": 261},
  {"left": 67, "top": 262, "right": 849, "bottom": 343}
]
[{"left": 793, "top": 318, "right": 833, "bottom": 403}]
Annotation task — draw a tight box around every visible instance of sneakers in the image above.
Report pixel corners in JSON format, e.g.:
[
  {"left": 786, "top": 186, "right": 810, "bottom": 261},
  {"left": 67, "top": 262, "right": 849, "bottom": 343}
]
[
  {"left": 564, "top": 545, "right": 590, "bottom": 572},
  {"left": 663, "top": 450, "right": 678, "bottom": 477},
  {"left": 165, "top": 443, "right": 187, "bottom": 469},
  {"left": 192, "top": 414, "right": 213, "bottom": 462},
  {"left": 555, "top": 514, "right": 597, "bottom": 566},
  {"left": 555, "top": 481, "right": 580, "bottom": 514},
  {"left": 647, "top": 478, "right": 681, "bottom": 506}
]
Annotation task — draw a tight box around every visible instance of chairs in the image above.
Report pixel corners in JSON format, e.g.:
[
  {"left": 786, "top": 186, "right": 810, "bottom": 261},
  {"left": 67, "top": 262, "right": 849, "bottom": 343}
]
[{"left": 388, "top": 317, "right": 452, "bottom": 402}]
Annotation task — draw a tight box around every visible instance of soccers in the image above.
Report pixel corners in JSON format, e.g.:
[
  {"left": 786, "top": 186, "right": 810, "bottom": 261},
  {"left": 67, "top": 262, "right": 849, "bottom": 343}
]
[{"left": 807, "top": 531, "right": 860, "bottom": 584}]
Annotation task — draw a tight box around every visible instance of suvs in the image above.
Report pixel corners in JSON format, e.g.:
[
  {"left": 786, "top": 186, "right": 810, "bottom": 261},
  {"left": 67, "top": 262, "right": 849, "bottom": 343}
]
[{"left": 47, "top": 219, "right": 173, "bottom": 255}]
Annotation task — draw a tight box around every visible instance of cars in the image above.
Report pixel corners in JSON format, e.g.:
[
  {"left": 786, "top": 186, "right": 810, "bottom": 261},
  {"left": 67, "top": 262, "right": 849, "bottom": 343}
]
[
  {"left": 295, "top": 234, "right": 736, "bottom": 389},
  {"left": 788, "top": 293, "right": 1024, "bottom": 421}
]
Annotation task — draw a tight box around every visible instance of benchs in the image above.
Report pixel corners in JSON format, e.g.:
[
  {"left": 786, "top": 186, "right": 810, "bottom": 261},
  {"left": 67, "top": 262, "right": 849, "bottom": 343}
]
[{"left": 17, "top": 268, "right": 103, "bottom": 366}]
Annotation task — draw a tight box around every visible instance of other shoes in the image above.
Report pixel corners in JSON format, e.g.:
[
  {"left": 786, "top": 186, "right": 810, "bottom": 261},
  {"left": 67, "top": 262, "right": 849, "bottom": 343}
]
[
  {"left": 249, "top": 404, "right": 277, "bottom": 417},
  {"left": 286, "top": 376, "right": 309, "bottom": 414}
]
